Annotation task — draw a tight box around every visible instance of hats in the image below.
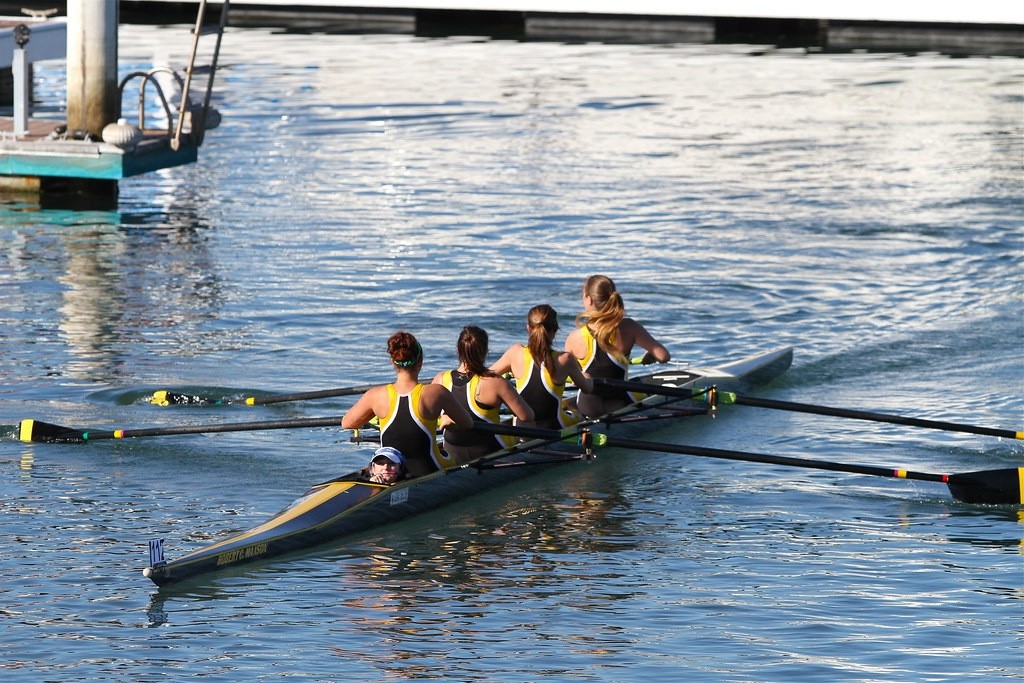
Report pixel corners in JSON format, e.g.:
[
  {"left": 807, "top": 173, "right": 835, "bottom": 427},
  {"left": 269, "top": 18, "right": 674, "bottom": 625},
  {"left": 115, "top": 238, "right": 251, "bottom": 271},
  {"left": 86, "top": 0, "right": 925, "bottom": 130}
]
[{"left": 371, "top": 447, "right": 403, "bottom": 465}]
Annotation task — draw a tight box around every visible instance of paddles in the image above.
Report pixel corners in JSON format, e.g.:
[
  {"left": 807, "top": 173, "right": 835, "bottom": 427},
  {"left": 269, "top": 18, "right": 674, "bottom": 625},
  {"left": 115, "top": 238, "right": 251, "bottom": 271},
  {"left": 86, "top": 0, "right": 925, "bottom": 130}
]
[
  {"left": 600, "top": 380, "right": 1024, "bottom": 441},
  {"left": 18, "top": 415, "right": 377, "bottom": 445},
  {"left": 154, "top": 369, "right": 512, "bottom": 405},
  {"left": 474, "top": 421, "right": 1022, "bottom": 505}
]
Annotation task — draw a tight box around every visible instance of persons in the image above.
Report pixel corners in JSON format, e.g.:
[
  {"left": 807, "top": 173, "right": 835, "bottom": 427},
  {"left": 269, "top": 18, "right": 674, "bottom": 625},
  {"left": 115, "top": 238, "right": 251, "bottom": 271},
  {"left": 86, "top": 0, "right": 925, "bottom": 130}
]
[
  {"left": 342, "top": 332, "right": 474, "bottom": 477},
  {"left": 362, "top": 447, "right": 408, "bottom": 486},
  {"left": 432, "top": 326, "right": 535, "bottom": 467},
  {"left": 488, "top": 304, "right": 593, "bottom": 442},
  {"left": 565, "top": 275, "right": 670, "bottom": 419}
]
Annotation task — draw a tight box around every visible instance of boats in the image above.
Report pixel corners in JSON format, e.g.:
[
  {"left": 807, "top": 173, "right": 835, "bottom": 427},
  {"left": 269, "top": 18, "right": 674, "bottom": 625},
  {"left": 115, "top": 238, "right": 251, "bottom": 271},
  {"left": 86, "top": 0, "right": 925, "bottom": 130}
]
[{"left": 143, "top": 345, "right": 793, "bottom": 587}]
